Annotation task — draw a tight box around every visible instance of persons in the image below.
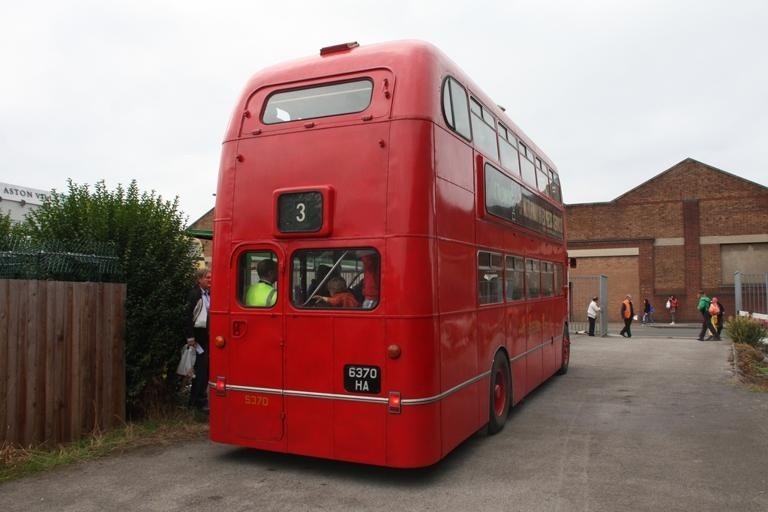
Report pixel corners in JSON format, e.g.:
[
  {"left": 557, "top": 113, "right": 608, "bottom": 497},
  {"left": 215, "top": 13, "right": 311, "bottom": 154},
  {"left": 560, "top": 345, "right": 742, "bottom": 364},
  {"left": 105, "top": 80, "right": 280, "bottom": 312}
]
[
  {"left": 667, "top": 295, "right": 678, "bottom": 322},
  {"left": 181, "top": 268, "right": 212, "bottom": 413},
  {"left": 696, "top": 291, "right": 725, "bottom": 341},
  {"left": 643, "top": 300, "right": 655, "bottom": 323},
  {"left": 586, "top": 296, "right": 600, "bottom": 336},
  {"left": 246, "top": 258, "right": 360, "bottom": 308},
  {"left": 620, "top": 294, "right": 635, "bottom": 337}
]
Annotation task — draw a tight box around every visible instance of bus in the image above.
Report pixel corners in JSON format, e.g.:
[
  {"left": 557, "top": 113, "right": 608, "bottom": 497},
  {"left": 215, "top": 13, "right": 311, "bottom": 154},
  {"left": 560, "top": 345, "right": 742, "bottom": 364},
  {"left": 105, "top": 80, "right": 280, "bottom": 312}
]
[{"left": 208, "top": 38, "right": 572, "bottom": 471}]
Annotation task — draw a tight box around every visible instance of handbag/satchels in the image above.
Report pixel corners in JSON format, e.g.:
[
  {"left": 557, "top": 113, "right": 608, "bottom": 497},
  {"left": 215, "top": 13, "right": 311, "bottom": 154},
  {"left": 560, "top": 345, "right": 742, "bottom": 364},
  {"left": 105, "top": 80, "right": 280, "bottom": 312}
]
[{"left": 176, "top": 344, "right": 197, "bottom": 378}]
